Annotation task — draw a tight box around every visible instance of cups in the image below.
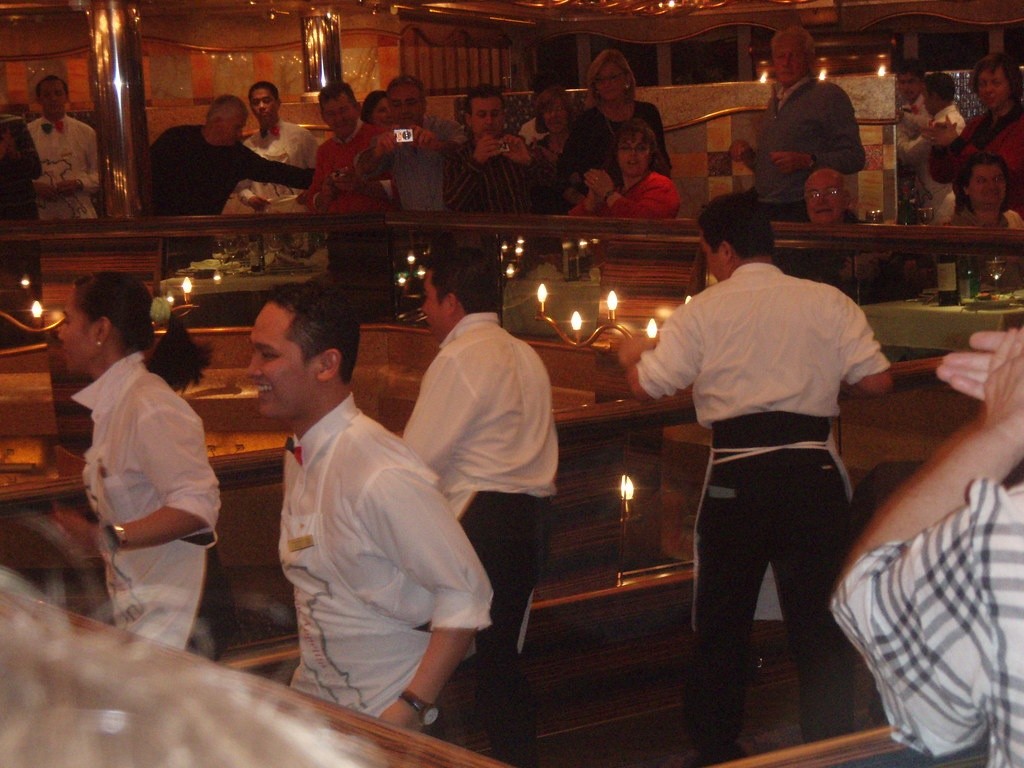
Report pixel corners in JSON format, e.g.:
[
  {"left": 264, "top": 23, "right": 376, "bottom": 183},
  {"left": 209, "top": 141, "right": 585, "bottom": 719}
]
[
  {"left": 865, "top": 210, "right": 885, "bottom": 224},
  {"left": 917, "top": 208, "right": 935, "bottom": 225}
]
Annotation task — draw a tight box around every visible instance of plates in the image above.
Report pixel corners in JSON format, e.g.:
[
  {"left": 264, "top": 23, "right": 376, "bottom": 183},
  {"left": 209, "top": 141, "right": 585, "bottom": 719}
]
[
  {"left": 961, "top": 294, "right": 1021, "bottom": 309},
  {"left": 266, "top": 194, "right": 298, "bottom": 203}
]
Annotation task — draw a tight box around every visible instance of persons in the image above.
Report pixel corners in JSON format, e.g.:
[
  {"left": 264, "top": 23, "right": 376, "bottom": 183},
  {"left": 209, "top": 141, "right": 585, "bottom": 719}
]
[
  {"left": 401, "top": 248, "right": 558, "bottom": 768},
  {"left": 0, "top": 89, "right": 42, "bottom": 303},
  {"left": 146, "top": 96, "right": 315, "bottom": 276},
  {"left": 26, "top": 74, "right": 101, "bottom": 220},
  {"left": 831, "top": 325, "right": 1023, "bottom": 768},
  {"left": 244, "top": 282, "right": 494, "bottom": 733},
  {"left": 727, "top": 25, "right": 901, "bottom": 305},
  {"left": 519, "top": 48, "right": 682, "bottom": 218},
  {"left": 619, "top": 193, "right": 893, "bottom": 768},
  {"left": 896, "top": 53, "right": 1024, "bottom": 293},
  {"left": 58, "top": 271, "right": 222, "bottom": 650},
  {"left": 239, "top": 75, "right": 556, "bottom": 213}
]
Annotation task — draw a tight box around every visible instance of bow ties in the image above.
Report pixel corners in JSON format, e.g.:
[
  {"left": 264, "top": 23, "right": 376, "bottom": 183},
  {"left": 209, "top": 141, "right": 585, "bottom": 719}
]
[
  {"left": 258, "top": 128, "right": 279, "bottom": 139},
  {"left": 285, "top": 436, "right": 303, "bottom": 465},
  {"left": 41, "top": 120, "right": 64, "bottom": 134},
  {"left": 909, "top": 105, "right": 917, "bottom": 111}
]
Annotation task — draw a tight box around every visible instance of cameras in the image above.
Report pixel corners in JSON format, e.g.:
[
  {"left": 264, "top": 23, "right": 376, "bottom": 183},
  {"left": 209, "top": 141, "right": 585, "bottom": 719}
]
[{"left": 393, "top": 129, "right": 413, "bottom": 142}]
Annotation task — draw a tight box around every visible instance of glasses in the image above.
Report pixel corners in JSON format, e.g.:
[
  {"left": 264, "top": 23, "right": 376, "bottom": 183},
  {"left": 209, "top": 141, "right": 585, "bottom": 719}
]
[
  {"left": 805, "top": 189, "right": 842, "bottom": 204},
  {"left": 618, "top": 142, "right": 647, "bottom": 154},
  {"left": 595, "top": 72, "right": 624, "bottom": 84}
]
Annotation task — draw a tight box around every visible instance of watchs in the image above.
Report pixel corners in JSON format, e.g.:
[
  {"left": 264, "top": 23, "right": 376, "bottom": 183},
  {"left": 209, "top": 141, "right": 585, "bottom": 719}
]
[
  {"left": 399, "top": 687, "right": 439, "bottom": 725},
  {"left": 806, "top": 151, "right": 817, "bottom": 168}
]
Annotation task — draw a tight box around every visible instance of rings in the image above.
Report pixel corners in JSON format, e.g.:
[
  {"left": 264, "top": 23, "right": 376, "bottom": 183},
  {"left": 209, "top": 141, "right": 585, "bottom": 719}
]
[{"left": 931, "top": 137, "right": 934, "bottom": 141}]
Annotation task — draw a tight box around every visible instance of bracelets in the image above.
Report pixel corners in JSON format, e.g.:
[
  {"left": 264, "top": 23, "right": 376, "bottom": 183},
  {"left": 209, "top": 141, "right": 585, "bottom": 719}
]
[
  {"left": 76, "top": 180, "right": 81, "bottom": 190},
  {"left": 604, "top": 189, "right": 616, "bottom": 203},
  {"left": 113, "top": 523, "right": 128, "bottom": 549},
  {"left": 104, "top": 524, "right": 120, "bottom": 547}
]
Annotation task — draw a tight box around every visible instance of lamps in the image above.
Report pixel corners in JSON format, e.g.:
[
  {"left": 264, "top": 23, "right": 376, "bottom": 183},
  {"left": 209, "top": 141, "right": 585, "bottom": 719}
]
[
  {"left": 531, "top": 282, "right": 663, "bottom": 350},
  {"left": 180, "top": 277, "right": 192, "bottom": 307},
  {"left": 31, "top": 297, "right": 43, "bottom": 326}
]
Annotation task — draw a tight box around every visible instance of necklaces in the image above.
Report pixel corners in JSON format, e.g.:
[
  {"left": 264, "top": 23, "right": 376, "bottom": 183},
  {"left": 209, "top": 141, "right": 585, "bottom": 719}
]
[
  {"left": 600, "top": 101, "right": 631, "bottom": 137},
  {"left": 619, "top": 171, "right": 651, "bottom": 195}
]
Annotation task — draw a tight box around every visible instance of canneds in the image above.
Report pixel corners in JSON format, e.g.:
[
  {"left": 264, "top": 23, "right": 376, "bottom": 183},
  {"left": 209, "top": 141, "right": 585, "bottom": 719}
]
[{"left": 866, "top": 209, "right": 884, "bottom": 224}]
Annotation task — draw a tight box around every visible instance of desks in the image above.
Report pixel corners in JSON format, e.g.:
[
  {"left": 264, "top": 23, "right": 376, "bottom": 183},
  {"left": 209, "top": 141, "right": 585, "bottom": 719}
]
[{"left": 854, "top": 293, "right": 1024, "bottom": 352}]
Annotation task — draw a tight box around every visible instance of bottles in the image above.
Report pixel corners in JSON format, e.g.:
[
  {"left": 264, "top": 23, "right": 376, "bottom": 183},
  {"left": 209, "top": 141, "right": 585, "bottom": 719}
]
[
  {"left": 249, "top": 222, "right": 265, "bottom": 271},
  {"left": 937, "top": 239, "right": 960, "bottom": 307}
]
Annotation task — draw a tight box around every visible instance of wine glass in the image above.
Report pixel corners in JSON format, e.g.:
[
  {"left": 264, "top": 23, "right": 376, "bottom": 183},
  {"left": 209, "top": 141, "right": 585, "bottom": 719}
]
[
  {"left": 984, "top": 255, "right": 1006, "bottom": 290},
  {"left": 212, "top": 231, "right": 322, "bottom": 277}
]
